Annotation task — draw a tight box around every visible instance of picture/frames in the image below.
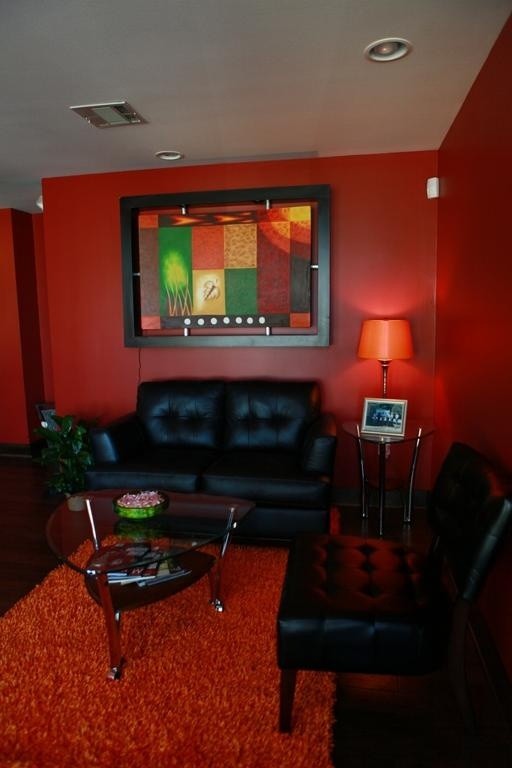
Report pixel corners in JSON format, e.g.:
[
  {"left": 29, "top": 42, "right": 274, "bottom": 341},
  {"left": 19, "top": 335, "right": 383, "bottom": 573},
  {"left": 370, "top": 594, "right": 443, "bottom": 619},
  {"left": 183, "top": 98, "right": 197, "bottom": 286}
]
[{"left": 360, "top": 397, "right": 408, "bottom": 437}]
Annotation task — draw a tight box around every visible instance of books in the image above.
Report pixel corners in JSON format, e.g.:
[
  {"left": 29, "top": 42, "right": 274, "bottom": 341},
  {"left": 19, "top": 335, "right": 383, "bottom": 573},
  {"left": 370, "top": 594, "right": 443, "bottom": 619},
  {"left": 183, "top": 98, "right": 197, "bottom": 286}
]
[{"left": 88, "top": 542, "right": 193, "bottom": 590}]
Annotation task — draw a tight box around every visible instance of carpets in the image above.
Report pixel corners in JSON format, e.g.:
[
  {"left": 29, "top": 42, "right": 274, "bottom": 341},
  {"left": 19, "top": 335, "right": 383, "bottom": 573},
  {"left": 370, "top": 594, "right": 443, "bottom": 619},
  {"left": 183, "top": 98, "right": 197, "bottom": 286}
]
[{"left": 0, "top": 534, "right": 342, "bottom": 768}]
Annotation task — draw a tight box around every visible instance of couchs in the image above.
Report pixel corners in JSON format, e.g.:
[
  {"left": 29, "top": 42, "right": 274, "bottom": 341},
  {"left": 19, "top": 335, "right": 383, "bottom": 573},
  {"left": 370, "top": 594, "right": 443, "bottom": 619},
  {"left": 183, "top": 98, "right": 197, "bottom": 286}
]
[{"left": 84, "top": 377, "right": 338, "bottom": 544}]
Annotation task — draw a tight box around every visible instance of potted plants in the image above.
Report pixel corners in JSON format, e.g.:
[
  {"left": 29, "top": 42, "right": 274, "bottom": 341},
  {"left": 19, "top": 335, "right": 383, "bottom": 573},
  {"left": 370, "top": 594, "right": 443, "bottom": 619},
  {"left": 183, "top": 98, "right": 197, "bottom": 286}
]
[{"left": 32, "top": 415, "right": 96, "bottom": 512}]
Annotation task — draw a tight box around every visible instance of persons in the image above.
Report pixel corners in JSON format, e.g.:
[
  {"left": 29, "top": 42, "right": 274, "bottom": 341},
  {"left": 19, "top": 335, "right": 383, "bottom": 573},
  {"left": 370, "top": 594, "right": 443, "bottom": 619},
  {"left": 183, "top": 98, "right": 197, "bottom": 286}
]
[{"left": 370, "top": 412, "right": 401, "bottom": 427}]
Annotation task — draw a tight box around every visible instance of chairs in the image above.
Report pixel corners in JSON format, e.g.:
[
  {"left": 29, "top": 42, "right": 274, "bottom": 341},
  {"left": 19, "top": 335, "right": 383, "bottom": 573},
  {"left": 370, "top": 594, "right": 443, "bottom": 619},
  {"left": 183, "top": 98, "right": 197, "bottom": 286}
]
[{"left": 275, "top": 440, "right": 510, "bottom": 733}]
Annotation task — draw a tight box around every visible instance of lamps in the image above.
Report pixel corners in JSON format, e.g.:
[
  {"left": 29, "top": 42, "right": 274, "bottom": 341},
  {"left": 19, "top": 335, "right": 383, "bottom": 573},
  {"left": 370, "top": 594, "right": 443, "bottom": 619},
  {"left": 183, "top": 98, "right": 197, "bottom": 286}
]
[{"left": 356, "top": 318, "right": 415, "bottom": 397}]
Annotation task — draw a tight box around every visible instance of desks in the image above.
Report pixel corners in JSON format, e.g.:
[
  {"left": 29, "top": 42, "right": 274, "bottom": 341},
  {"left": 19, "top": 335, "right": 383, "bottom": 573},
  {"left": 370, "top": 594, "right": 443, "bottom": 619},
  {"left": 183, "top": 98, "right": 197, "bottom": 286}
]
[{"left": 342, "top": 419, "right": 435, "bottom": 535}]
